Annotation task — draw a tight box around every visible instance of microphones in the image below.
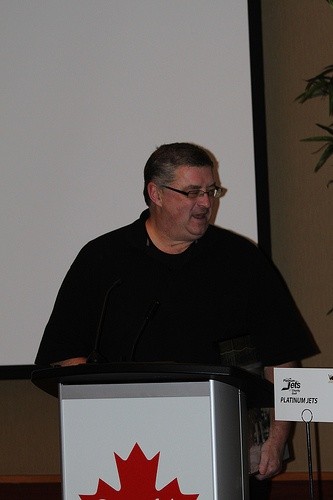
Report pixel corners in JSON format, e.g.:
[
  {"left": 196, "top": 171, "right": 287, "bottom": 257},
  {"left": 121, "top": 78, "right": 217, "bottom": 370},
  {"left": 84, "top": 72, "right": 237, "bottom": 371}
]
[
  {"left": 86, "top": 276, "right": 122, "bottom": 362},
  {"left": 129, "top": 292, "right": 163, "bottom": 363}
]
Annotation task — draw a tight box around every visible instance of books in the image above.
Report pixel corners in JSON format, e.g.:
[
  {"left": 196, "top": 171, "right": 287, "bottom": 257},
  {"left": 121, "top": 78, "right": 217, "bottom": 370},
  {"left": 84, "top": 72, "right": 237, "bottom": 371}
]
[{"left": 217, "top": 334, "right": 295, "bottom": 477}]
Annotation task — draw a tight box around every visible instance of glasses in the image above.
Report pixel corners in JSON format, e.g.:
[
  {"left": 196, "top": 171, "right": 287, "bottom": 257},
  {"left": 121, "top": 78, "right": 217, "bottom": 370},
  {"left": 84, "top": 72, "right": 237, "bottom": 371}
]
[{"left": 155, "top": 184, "right": 227, "bottom": 200}]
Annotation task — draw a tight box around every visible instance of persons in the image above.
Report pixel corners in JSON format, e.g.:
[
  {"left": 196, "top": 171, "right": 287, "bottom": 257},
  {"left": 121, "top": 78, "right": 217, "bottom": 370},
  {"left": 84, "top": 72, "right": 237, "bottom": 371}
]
[{"left": 34, "top": 142, "right": 320, "bottom": 500}]
